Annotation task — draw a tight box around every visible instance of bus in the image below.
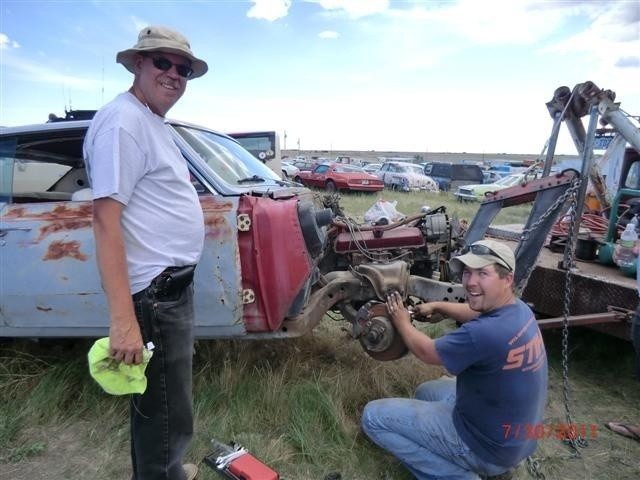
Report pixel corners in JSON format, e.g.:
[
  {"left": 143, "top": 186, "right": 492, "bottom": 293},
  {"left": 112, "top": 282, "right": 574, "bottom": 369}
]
[{"left": 229, "top": 131, "right": 281, "bottom": 178}]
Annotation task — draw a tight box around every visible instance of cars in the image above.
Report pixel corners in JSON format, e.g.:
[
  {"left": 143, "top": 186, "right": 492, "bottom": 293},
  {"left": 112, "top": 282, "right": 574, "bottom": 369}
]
[
  {"left": 282, "top": 156, "right": 438, "bottom": 193},
  {"left": 455, "top": 175, "right": 532, "bottom": 203}
]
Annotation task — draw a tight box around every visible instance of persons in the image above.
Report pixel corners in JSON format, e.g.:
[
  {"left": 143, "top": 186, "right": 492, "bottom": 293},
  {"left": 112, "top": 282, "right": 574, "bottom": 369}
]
[
  {"left": 604, "top": 238, "right": 640, "bottom": 442},
  {"left": 359, "top": 237, "right": 548, "bottom": 479},
  {"left": 83, "top": 25, "right": 209, "bottom": 480}
]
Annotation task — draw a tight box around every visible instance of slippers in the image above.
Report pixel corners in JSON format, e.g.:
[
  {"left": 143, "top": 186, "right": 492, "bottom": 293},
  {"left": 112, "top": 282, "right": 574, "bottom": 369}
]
[{"left": 604, "top": 420, "right": 640, "bottom": 442}]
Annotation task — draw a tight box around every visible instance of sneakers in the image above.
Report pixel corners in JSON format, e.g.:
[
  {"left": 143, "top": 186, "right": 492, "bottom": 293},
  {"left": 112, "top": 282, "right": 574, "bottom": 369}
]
[{"left": 181, "top": 463, "right": 199, "bottom": 480}]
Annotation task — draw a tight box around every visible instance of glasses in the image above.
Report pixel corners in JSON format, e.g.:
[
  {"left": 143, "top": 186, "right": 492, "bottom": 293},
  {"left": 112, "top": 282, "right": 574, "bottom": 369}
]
[
  {"left": 460, "top": 244, "right": 512, "bottom": 271},
  {"left": 140, "top": 52, "right": 194, "bottom": 78}
]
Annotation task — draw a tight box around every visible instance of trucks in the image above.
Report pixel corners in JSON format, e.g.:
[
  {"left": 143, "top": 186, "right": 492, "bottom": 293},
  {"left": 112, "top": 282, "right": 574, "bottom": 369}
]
[{"left": 444, "top": 81, "right": 640, "bottom": 344}]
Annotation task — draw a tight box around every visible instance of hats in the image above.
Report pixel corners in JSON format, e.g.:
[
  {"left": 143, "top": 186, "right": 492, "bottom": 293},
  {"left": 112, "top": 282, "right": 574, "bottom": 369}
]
[
  {"left": 448, "top": 240, "right": 517, "bottom": 274},
  {"left": 117, "top": 26, "right": 208, "bottom": 79}
]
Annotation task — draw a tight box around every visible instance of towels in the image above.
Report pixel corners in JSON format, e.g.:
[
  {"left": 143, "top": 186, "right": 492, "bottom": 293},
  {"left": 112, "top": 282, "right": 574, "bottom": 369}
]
[{"left": 88, "top": 337, "right": 155, "bottom": 397}]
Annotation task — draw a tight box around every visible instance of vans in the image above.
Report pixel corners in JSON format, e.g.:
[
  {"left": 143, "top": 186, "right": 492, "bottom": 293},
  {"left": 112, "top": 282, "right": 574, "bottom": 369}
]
[{"left": 424, "top": 162, "right": 485, "bottom": 192}]
